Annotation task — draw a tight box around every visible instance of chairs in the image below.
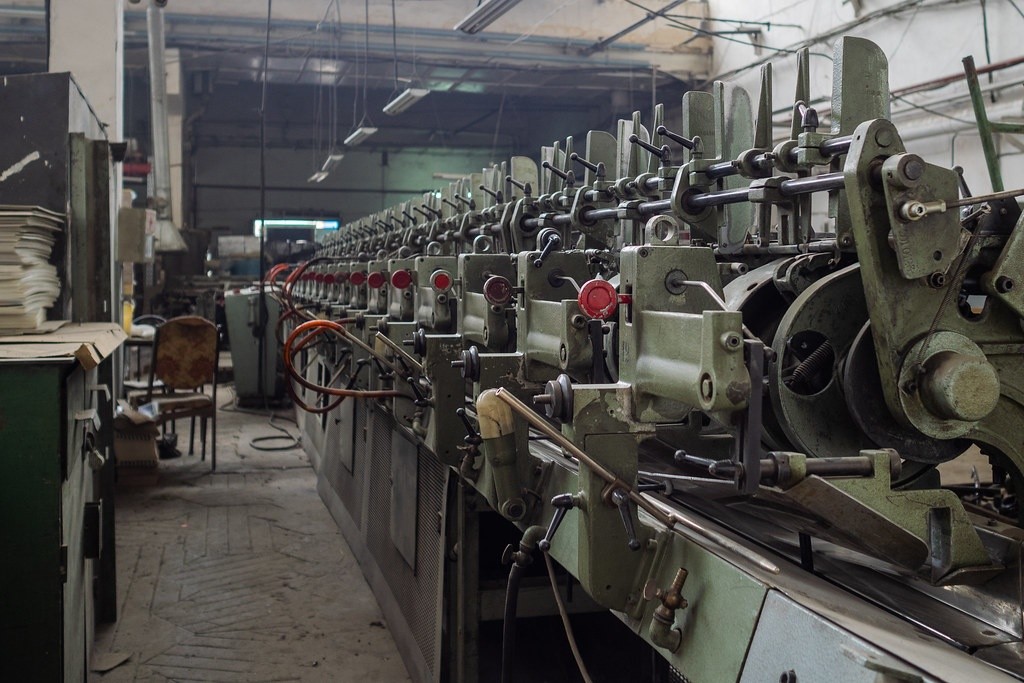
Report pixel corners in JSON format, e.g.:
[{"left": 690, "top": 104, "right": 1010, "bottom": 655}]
[{"left": 123, "top": 314, "right": 221, "bottom": 472}]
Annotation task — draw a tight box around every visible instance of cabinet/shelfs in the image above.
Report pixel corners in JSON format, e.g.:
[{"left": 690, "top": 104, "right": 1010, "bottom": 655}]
[{"left": 0, "top": 356, "right": 111, "bottom": 683}]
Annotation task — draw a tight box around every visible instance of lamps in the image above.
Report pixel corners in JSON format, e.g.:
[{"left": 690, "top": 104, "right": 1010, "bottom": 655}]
[
  {"left": 453, "top": 0, "right": 521, "bottom": 35},
  {"left": 307, "top": 0, "right": 431, "bottom": 183}
]
